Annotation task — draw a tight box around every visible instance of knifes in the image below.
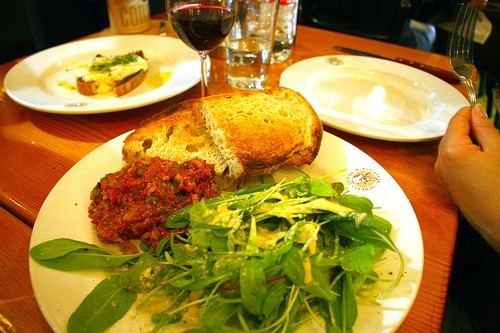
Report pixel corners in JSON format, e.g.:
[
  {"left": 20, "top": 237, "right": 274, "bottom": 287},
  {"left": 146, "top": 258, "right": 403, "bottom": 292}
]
[{"left": 334, "top": 45, "right": 460, "bottom": 85}]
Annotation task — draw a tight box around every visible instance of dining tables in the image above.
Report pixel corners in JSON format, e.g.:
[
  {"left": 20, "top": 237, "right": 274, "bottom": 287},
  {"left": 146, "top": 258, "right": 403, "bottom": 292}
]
[{"left": 0, "top": 11, "right": 479, "bottom": 333}]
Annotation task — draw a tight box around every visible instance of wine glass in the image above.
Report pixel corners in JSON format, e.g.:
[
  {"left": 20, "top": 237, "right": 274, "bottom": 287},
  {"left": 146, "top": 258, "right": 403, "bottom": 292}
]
[{"left": 165, "top": 0, "right": 239, "bottom": 98}]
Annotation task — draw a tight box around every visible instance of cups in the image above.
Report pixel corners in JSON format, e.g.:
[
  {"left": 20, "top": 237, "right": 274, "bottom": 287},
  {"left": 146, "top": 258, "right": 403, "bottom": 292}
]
[{"left": 224, "top": 0, "right": 280, "bottom": 91}]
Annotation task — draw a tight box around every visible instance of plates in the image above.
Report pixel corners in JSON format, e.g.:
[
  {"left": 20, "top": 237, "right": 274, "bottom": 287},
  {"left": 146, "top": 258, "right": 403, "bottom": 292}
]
[
  {"left": 278, "top": 54, "right": 471, "bottom": 142},
  {"left": 3, "top": 35, "right": 211, "bottom": 115},
  {"left": 29, "top": 128, "right": 425, "bottom": 333}
]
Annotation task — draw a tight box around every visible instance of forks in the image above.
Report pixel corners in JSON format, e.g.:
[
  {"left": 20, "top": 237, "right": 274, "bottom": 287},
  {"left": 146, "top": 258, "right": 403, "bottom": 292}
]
[{"left": 448, "top": 2, "right": 482, "bottom": 111}]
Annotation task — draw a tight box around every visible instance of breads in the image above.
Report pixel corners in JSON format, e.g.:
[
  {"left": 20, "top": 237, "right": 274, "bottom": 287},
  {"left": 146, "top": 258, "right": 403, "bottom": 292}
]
[
  {"left": 122, "top": 87, "right": 324, "bottom": 178},
  {"left": 76, "top": 50, "right": 146, "bottom": 96}
]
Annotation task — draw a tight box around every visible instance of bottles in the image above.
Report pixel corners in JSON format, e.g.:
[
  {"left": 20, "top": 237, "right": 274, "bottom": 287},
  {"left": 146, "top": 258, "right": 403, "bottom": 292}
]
[
  {"left": 269, "top": 0, "right": 300, "bottom": 64},
  {"left": 107, "top": 0, "right": 152, "bottom": 34}
]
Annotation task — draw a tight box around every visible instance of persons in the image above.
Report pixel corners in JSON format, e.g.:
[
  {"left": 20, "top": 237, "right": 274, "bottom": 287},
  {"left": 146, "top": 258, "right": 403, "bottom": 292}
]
[
  {"left": 310, "top": 0, "right": 436, "bottom": 51},
  {"left": 435, "top": 103, "right": 500, "bottom": 333}
]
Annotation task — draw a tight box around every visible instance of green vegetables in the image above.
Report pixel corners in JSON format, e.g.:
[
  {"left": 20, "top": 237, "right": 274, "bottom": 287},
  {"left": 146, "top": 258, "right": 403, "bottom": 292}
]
[
  {"left": 29, "top": 167, "right": 403, "bottom": 333},
  {"left": 90, "top": 54, "right": 138, "bottom": 71}
]
